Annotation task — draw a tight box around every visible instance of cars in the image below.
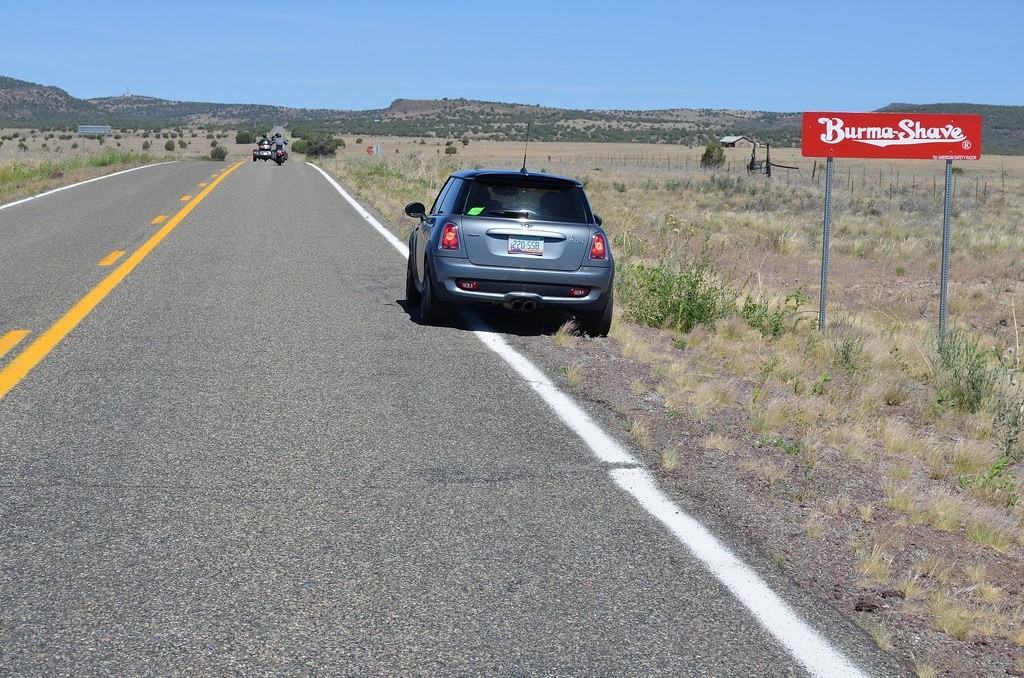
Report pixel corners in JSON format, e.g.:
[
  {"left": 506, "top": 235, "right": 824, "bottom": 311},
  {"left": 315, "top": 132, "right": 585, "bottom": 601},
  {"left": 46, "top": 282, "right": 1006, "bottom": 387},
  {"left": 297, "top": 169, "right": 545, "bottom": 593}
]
[{"left": 405, "top": 168, "right": 614, "bottom": 337}]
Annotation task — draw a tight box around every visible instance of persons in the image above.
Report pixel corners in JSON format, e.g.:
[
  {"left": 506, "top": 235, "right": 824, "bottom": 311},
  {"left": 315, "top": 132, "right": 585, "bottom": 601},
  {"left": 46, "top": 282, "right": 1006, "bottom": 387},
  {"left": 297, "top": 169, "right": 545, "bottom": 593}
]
[
  {"left": 257, "top": 133, "right": 288, "bottom": 153},
  {"left": 477, "top": 188, "right": 502, "bottom": 209}
]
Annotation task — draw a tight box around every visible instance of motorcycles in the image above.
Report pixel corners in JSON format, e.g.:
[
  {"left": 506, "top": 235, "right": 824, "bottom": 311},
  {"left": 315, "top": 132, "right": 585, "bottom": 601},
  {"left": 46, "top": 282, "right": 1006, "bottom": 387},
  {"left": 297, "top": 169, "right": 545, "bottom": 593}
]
[{"left": 253, "top": 136, "right": 289, "bottom": 166}]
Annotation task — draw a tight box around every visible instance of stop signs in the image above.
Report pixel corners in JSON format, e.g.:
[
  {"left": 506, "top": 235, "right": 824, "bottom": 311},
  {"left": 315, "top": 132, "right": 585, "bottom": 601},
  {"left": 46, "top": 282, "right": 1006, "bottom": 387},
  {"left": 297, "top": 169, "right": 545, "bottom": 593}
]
[{"left": 367, "top": 146, "right": 374, "bottom": 154}]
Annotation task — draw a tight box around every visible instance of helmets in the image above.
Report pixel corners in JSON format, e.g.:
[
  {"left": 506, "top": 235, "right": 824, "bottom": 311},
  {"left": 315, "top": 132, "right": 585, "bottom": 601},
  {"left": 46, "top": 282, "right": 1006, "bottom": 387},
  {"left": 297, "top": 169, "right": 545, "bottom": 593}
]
[
  {"left": 263, "top": 134, "right": 267, "bottom": 138},
  {"left": 277, "top": 133, "right": 281, "bottom": 138}
]
